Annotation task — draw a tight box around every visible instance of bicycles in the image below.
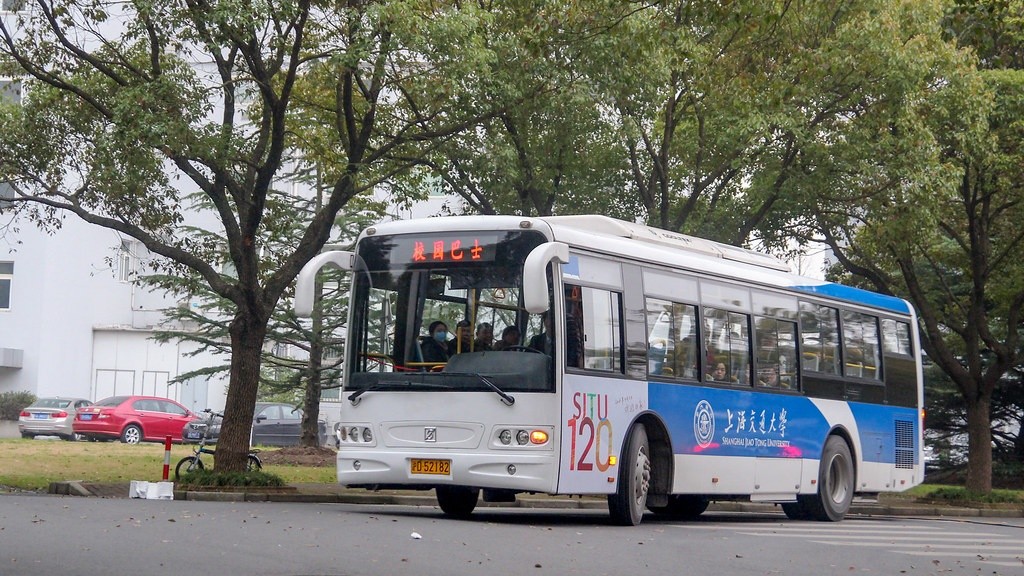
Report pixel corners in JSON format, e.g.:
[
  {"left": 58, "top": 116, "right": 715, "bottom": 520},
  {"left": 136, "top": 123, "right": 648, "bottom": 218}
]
[{"left": 175, "top": 409, "right": 266, "bottom": 481}]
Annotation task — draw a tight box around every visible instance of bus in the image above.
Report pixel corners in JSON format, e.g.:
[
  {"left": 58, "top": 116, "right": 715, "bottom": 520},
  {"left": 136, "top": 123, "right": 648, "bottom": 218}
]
[{"left": 293, "top": 214, "right": 926, "bottom": 528}]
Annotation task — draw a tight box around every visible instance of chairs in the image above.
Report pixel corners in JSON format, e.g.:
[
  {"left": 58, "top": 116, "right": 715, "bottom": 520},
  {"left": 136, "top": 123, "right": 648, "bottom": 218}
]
[
  {"left": 707, "top": 374, "right": 714, "bottom": 381},
  {"left": 663, "top": 367, "right": 673, "bottom": 376},
  {"left": 732, "top": 376, "right": 739, "bottom": 384},
  {"left": 804, "top": 353, "right": 818, "bottom": 372},
  {"left": 846, "top": 362, "right": 862, "bottom": 377},
  {"left": 416, "top": 336, "right": 427, "bottom": 372}
]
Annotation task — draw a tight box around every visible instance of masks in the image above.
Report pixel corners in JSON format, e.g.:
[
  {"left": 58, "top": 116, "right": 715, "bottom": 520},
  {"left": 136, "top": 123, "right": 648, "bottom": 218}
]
[{"left": 433, "top": 332, "right": 446, "bottom": 342}]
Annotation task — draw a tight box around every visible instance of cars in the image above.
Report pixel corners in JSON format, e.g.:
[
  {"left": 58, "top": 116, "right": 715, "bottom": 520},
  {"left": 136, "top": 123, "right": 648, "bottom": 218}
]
[
  {"left": 71, "top": 396, "right": 202, "bottom": 447},
  {"left": 183, "top": 402, "right": 329, "bottom": 447},
  {"left": 19, "top": 397, "right": 95, "bottom": 441}
]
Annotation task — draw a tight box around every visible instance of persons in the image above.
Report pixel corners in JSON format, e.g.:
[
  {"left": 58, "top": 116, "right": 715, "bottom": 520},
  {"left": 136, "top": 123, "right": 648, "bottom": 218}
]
[
  {"left": 525, "top": 311, "right": 554, "bottom": 357},
  {"left": 421, "top": 321, "right": 454, "bottom": 373},
  {"left": 711, "top": 361, "right": 777, "bottom": 386},
  {"left": 448, "top": 321, "right": 520, "bottom": 353}
]
